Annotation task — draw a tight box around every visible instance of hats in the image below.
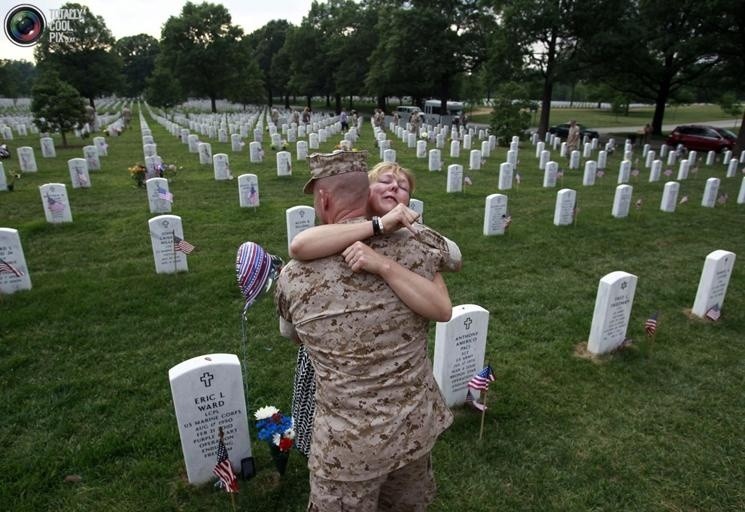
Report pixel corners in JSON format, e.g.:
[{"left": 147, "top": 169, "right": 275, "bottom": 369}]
[{"left": 303, "top": 151, "right": 368, "bottom": 194}]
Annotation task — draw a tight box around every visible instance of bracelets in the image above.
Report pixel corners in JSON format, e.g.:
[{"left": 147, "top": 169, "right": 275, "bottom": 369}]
[
  {"left": 372, "top": 215, "right": 380, "bottom": 236},
  {"left": 378, "top": 216, "right": 385, "bottom": 236}
]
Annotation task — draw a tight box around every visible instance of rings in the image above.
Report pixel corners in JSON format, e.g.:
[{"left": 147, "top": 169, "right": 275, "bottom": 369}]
[{"left": 351, "top": 258, "right": 358, "bottom": 264}]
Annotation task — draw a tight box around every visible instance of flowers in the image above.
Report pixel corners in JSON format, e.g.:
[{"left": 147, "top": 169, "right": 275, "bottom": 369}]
[
  {"left": 253, "top": 405, "right": 296, "bottom": 475},
  {"left": 154, "top": 160, "right": 167, "bottom": 178},
  {"left": 127, "top": 165, "right": 145, "bottom": 186}
]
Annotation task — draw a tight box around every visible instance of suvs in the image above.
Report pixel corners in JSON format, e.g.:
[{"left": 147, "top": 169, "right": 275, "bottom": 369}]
[{"left": 668, "top": 125, "right": 739, "bottom": 154}]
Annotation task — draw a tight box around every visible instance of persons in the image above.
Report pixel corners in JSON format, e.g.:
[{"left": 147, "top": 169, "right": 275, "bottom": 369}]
[
  {"left": 340, "top": 107, "right": 361, "bottom": 137},
  {"left": 273, "top": 152, "right": 462, "bottom": 512},
  {"left": 566, "top": 119, "right": 581, "bottom": 155},
  {"left": 123, "top": 104, "right": 133, "bottom": 130},
  {"left": 644, "top": 124, "right": 653, "bottom": 144},
  {"left": 291, "top": 161, "right": 452, "bottom": 460},
  {"left": 269, "top": 106, "right": 279, "bottom": 126},
  {"left": 290, "top": 106, "right": 311, "bottom": 127},
  {"left": 374, "top": 109, "right": 426, "bottom": 131}
]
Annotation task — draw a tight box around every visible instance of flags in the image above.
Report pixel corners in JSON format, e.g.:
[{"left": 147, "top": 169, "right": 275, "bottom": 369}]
[
  {"left": 156, "top": 186, "right": 176, "bottom": 203},
  {"left": 248, "top": 188, "right": 258, "bottom": 206},
  {"left": 75, "top": 170, "right": 88, "bottom": 188},
  {"left": 212, "top": 439, "right": 241, "bottom": 494},
  {"left": 467, "top": 367, "right": 496, "bottom": 400},
  {"left": 170, "top": 235, "right": 196, "bottom": 254},
  {"left": 0, "top": 258, "right": 25, "bottom": 278},
  {"left": 644, "top": 311, "right": 660, "bottom": 338},
  {"left": 47, "top": 197, "right": 65, "bottom": 213}
]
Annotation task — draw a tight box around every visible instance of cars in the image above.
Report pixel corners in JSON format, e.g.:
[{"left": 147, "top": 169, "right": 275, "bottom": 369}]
[{"left": 551, "top": 123, "right": 599, "bottom": 141}]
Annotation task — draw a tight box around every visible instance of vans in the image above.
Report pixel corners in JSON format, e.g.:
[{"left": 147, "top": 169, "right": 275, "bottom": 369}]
[{"left": 395, "top": 100, "right": 469, "bottom": 124}]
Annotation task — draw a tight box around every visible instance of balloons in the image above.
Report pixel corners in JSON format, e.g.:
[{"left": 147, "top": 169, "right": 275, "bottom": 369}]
[{"left": 235, "top": 241, "right": 285, "bottom": 312}]
